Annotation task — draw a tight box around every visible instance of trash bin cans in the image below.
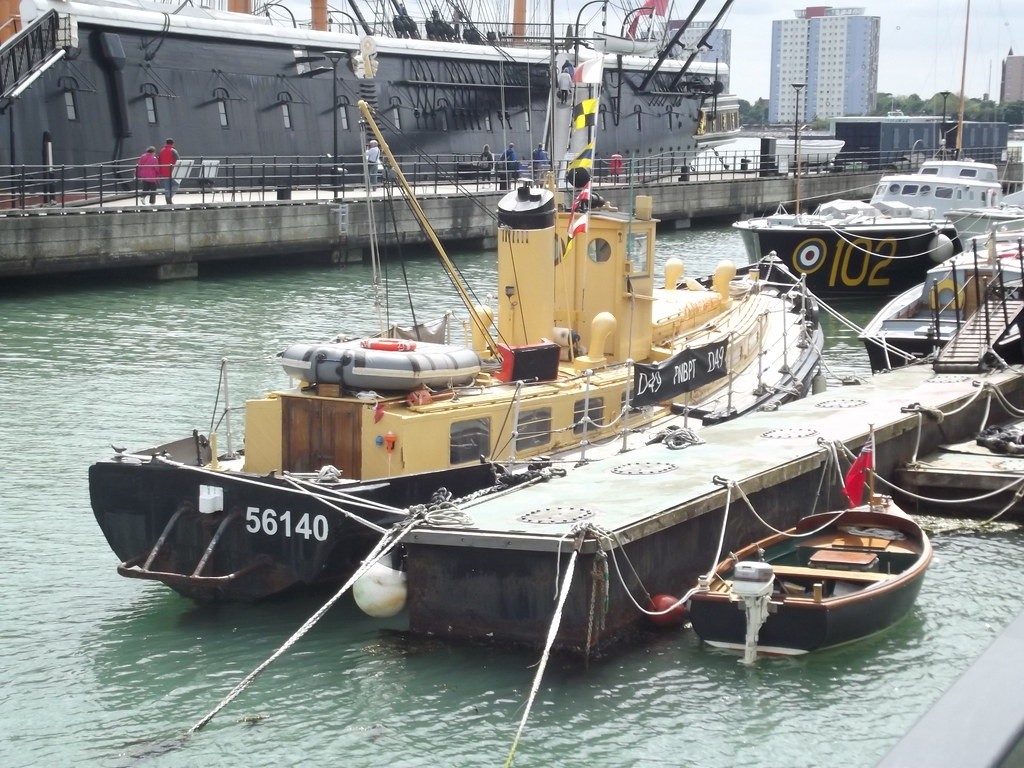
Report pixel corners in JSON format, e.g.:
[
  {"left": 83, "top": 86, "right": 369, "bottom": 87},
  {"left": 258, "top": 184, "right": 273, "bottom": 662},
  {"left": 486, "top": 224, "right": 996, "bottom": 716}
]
[
  {"left": 741, "top": 159, "right": 748, "bottom": 170},
  {"left": 277, "top": 185, "right": 291, "bottom": 199},
  {"left": 681, "top": 166, "right": 690, "bottom": 181}
]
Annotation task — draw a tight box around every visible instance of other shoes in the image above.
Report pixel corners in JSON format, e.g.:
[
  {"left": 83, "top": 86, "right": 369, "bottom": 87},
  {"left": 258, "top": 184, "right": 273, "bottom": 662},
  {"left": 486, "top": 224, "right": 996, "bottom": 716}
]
[
  {"left": 489, "top": 183, "right": 492, "bottom": 188},
  {"left": 140, "top": 197, "right": 145, "bottom": 205},
  {"left": 149, "top": 203, "right": 156, "bottom": 205},
  {"left": 482, "top": 185, "right": 485, "bottom": 189}
]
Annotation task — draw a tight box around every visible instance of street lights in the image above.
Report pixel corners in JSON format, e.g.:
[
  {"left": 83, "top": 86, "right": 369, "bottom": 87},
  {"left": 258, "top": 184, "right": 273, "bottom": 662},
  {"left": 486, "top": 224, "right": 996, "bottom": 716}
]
[
  {"left": 940, "top": 91, "right": 950, "bottom": 157},
  {"left": 791, "top": 83, "right": 806, "bottom": 176},
  {"left": 323, "top": 49, "right": 346, "bottom": 200}
]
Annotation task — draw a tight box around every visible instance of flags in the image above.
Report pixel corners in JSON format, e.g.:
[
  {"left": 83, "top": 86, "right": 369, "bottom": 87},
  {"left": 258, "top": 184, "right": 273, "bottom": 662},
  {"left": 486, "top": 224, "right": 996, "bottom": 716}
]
[
  {"left": 573, "top": 57, "right": 602, "bottom": 83},
  {"left": 572, "top": 99, "right": 598, "bottom": 129},
  {"left": 568, "top": 143, "right": 593, "bottom": 169},
  {"left": 563, "top": 181, "right": 590, "bottom": 257},
  {"left": 841, "top": 432, "right": 876, "bottom": 506}
]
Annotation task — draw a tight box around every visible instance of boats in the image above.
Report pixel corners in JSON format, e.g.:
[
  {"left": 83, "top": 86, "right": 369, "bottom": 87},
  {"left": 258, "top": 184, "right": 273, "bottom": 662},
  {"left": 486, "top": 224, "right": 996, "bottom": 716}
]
[
  {"left": 685, "top": 420, "right": 936, "bottom": 657},
  {"left": 0, "top": 1, "right": 1024, "bottom": 613}
]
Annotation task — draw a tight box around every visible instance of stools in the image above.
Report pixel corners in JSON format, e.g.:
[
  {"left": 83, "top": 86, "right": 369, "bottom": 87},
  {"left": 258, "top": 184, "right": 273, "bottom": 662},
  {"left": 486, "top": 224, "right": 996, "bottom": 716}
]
[{"left": 494, "top": 337, "right": 561, "bottom": 384}]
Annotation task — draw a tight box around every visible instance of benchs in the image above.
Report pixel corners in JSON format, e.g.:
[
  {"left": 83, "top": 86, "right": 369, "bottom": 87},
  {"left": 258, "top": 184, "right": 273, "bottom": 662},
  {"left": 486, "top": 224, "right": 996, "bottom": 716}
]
[{"left": 769, "top": 565, "right": 893, "bottom": 582}]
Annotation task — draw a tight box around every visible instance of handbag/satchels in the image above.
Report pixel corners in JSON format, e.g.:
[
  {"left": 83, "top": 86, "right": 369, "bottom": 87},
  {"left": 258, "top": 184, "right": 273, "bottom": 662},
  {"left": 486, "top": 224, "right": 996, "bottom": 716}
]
[{"left": 367, "top": 161, "right": 375, "bottom": 167}]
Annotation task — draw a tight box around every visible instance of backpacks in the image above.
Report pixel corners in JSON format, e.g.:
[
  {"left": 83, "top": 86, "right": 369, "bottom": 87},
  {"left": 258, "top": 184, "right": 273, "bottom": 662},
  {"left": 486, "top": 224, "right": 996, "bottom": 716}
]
[
  {"left": 481, "top": 153, "right": 488, "bottom": 168},
  {"left": 532, "top": 149, "right": 542, "bottom": 163},
  {"left": 504, "top": 150, "right": 513, "bottom": 164}
]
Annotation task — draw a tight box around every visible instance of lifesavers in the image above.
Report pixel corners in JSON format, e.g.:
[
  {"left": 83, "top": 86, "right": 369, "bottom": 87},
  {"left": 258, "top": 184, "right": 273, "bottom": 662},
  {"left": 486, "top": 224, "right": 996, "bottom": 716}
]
[
  {"left": 361, "top": 338, "right": 416, "bottom": 352},
  {"left": 929, "top": 278, "right": 965, "bottom": 310}
]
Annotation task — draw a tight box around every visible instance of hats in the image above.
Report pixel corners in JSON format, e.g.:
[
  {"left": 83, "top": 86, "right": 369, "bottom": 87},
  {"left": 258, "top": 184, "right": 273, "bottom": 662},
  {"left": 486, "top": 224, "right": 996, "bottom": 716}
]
[{"left": 166, "top": 138, "right": 175, "bottom": 144}]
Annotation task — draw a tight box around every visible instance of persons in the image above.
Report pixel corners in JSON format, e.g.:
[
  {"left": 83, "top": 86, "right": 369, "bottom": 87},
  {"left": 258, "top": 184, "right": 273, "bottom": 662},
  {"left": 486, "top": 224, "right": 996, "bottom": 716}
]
[
  {"left": 138, "top": 145, "right": 160, "bottom": 204},
  {"left": 480, "top": 144, "right": 494, "bottom": 189},
  {"left": 562, "top": 60, "right": 574, "bottom": 93},
  {"left": 400, "top": 3, "right": 406, "bottom": 15},
  {"left": 500, "top": 142, "right": 516, "bottom": 189},
  {"left": 533, "top": 143, "right": 549, "bottom": 188},
  {"left": 159, "top": 138, "right": 180, "bottom": 204},
  {"left": 454, "top": 6, "right": 460, "bottom": 40},
  {"left": 364, "top": 140, "right": 380, "bottom": 191},
  {"left": 558, "top": 68, "right": 572, "bottom": 104},
  {"left": 611, "top": 152, "right": 623, "bottom": 183}
]
